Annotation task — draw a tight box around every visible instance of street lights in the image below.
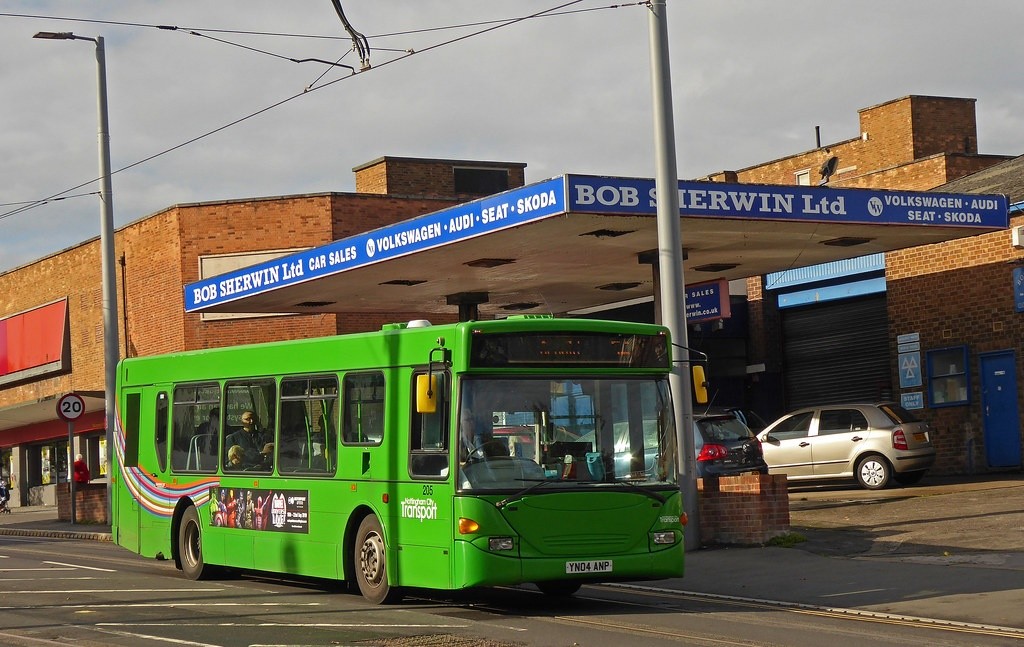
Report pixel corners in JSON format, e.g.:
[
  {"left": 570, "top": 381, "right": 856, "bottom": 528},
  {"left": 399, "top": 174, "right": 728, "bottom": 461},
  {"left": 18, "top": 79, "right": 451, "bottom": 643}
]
[{"left": 33, "top": 31, "right": 121, "bottom": 527}]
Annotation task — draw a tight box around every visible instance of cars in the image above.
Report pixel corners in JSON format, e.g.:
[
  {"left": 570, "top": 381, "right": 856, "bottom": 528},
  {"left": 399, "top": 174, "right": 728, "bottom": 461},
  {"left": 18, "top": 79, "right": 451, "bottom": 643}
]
[
  {"left": 493, "top": 425, "right": 581, "bottom": 457},
  {"left": 756, "top": 401, "right": 937, "bottom": 490},
  {"left": 574, "top": 413, "right": 768, "bottom": 491}
]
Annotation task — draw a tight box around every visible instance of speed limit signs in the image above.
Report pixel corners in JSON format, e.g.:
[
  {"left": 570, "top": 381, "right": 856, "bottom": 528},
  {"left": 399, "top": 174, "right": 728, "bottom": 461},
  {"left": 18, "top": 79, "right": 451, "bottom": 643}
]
[{"left": 58, "top": 393, "right": 86, "bottom": 421}]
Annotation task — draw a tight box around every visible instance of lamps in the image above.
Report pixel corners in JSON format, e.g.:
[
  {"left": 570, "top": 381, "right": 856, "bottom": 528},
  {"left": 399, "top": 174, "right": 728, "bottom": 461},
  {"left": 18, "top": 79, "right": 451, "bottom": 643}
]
[{"left": 816, "top": 157, "right": 838, "bottom": 187}]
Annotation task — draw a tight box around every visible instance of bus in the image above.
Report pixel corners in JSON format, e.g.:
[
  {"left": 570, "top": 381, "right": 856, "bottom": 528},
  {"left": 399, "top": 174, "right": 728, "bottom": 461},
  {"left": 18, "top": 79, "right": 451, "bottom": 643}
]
[{"left": 110, "top": 314, "right": 711, "bottom": 605}]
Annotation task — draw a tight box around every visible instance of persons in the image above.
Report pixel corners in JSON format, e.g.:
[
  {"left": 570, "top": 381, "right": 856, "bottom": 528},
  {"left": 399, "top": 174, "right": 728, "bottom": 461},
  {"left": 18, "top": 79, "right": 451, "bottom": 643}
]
[
  {"left": 459, "top": 409, "right": 485, "bottom": 462},
  {"left": 225, "top": 411, "right": 275, "bottom": 471},
  {"left": 196, "top": 407, "right": 231, "bottom": 454},
  {"left": 74, "top": 454, "right": 90, "bottom": 484}
]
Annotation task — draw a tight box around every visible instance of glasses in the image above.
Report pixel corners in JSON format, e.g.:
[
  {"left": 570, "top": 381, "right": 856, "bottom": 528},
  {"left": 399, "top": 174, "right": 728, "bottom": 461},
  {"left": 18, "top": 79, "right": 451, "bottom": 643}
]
[{"left": 462, "top": 418, "right": 475, "bottom": 422}]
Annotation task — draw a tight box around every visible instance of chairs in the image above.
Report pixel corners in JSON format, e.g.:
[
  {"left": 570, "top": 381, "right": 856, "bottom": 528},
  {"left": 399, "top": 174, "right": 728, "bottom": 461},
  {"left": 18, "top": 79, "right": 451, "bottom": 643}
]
[{"left": 173, "top": 422, "right": 368, "bottom": 472}]
